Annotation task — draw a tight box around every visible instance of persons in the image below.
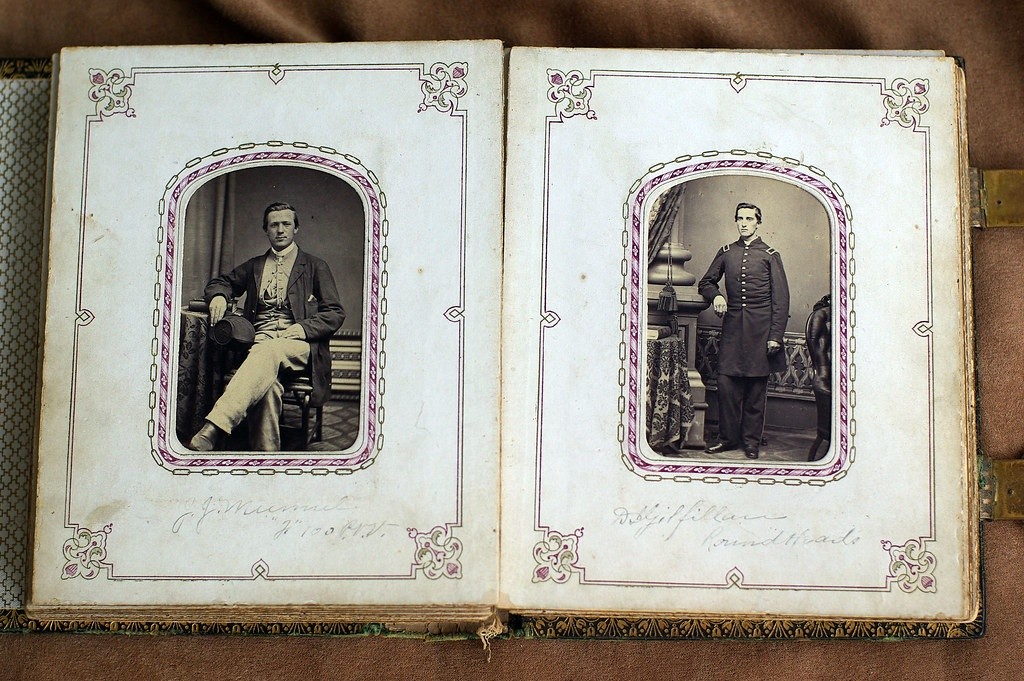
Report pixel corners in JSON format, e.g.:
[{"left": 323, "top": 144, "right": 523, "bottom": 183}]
[
  {"left": 696, "top": 203, "right": 791, "bottom": 455},
  {"left": 190, "top": 202, "right": 346, "bottom": 449}
]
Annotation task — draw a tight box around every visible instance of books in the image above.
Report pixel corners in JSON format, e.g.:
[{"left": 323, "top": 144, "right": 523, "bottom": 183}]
[{"left": 0, "top": 39, "right": 1024, "bottom": 645}]
